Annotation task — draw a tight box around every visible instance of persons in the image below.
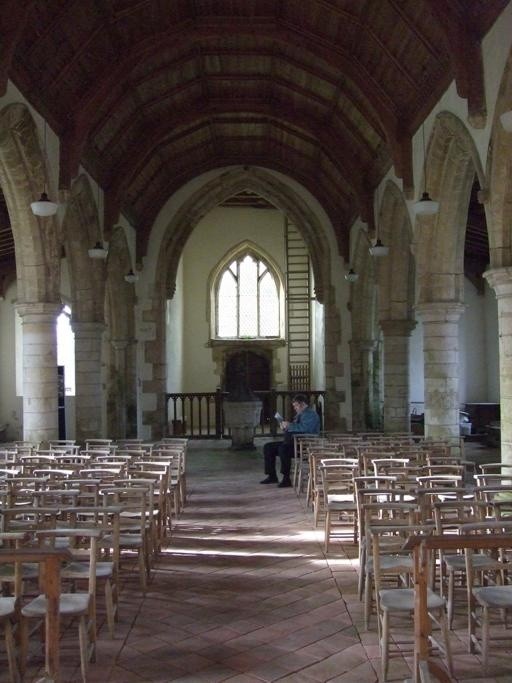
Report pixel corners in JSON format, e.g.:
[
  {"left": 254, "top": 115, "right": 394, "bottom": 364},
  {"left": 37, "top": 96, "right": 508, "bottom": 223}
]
[{"left": 259, "top": 393, "right": 321, "bottom": 488}]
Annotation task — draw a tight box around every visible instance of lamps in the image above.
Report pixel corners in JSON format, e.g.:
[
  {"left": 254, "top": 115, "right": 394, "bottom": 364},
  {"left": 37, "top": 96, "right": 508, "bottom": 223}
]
[
  {"left": 411, "top": 123, "right": 440, "bottom": 216},
  {"left": 30, "top": 119, "right": 59, "bottom": 217},
  {"left": 88, "top": 186, "right": 108, "bottom": 259},
  {"left": 367, "top": 188, "right": 390, "bottom": 255},
  {"left": 124, "top": 226, "right": 139, "bottom": 284},
  {"left": 344, "top": 229, "right": 360, "bottom": 282}
]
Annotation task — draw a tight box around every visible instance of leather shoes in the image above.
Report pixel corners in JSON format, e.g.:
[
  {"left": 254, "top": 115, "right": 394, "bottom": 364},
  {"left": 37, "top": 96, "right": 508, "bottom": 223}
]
[
  {"left": 260, "top": 476, "right": 278, "bottom": 484},
  {"left": 277, "top": 479, "right": 291, "bottom": 487}
]
